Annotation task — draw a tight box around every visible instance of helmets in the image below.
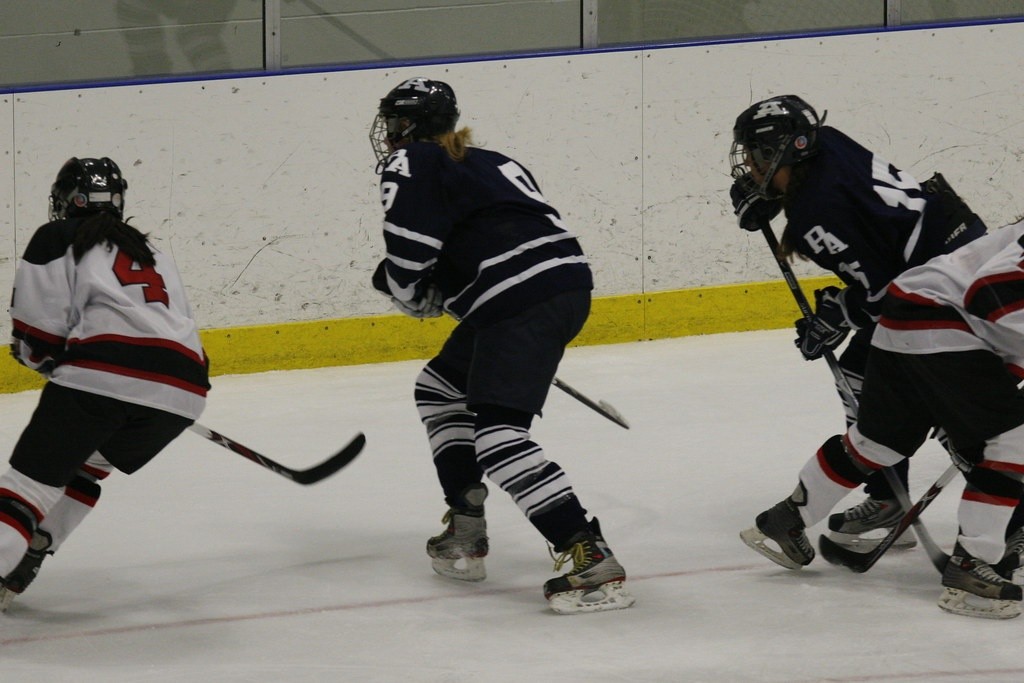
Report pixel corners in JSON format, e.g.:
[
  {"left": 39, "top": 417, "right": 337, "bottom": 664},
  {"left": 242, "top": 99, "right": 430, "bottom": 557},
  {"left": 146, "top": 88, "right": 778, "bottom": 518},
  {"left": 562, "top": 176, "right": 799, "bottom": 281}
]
[
  {"left": 47, "top": 156, "right": 128, "bottom": 222},
  {"left": 728, "top": 94, "right": 828, "bottom": 201},
  {"left": 369, "top": 77, "right": 460, "bottom": 167}
]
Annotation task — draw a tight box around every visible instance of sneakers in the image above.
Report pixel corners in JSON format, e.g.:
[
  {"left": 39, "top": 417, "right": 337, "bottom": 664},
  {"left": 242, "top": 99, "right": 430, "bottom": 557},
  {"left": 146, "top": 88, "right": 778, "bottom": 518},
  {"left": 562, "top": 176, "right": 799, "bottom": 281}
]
[
  {"left": 987, "top": 527, "right": 1024, "bottom": 585},
  {"left": 0, "top": 527, "right": 54, "bottom": 612},
  {"left": 827, "top": 487, "right": 917, "bottom": 552},
  {"left": 426, "top": 483, "right": 489, "bottom": 581},
  {"left": 739, "top": 480, "right": 816, "bottom": 571},
  {"left": 542, "top": 516, "right": 636, "bottom": 614},
  {"left": 938, "top": 524, "right": 1023, "bottom": 621}
]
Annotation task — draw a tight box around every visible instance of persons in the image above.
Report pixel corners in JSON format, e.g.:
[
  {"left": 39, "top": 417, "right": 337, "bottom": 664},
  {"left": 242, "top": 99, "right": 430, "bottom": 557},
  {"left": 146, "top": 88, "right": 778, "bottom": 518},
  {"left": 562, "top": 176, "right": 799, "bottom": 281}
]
[
  {"left": 739, "top": 215, "right": 1024, "bottom": 618},
  {"left": 729, "top": 96, "right": 1024, "bottom": 584},
  {"left": 372, "top": 76, "right": 636, "bottom": 615},
  {"left": 0, "top": 158, "right": 210, "bottom": 610}
]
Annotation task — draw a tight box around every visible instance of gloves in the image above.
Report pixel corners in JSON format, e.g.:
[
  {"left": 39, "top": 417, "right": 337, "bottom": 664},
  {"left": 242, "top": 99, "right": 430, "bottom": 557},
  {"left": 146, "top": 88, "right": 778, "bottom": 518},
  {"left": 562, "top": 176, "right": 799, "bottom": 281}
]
[
  {"left": 794, "top": 285, "right": 872, "bottom": 360},
  {"left": 729, "top": 170, "right": 786, "bottom": 232},
  {"left": 371, "top": 257, "right": 445, "bottom": 319}
]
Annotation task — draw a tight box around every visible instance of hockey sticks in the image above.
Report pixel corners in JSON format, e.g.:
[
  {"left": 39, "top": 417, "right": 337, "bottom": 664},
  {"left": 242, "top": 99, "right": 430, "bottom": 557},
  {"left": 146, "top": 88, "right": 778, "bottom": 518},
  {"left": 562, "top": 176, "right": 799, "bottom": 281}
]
[
  {"left": 760, "top": 222, "right": 1021, "bottom": 578},
  {"left": 444, "top": 309, "right": 631, "bottom": 431},
  {"left": 816, "top": 463, "right": 960, "bottom": 575},
  {"left": 188, "top": 422, "right": 367, "bottom": 486}
]
